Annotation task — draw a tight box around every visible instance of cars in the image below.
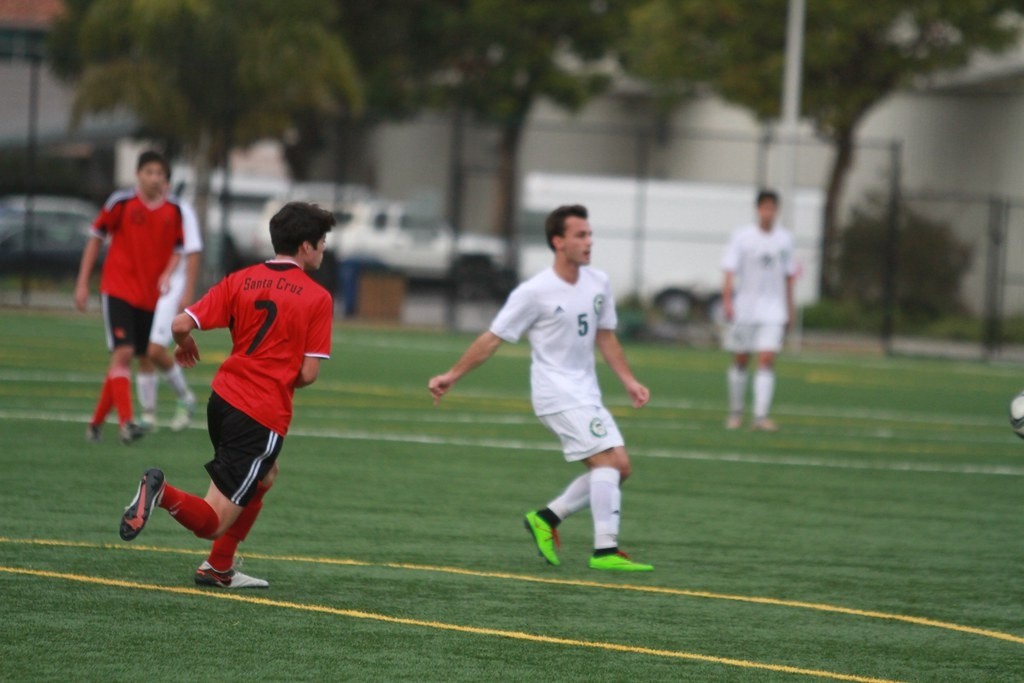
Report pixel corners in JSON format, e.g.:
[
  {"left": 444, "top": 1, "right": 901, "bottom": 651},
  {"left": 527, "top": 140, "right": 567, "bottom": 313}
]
[
  {"left": 194, "top": 162, "right": 824, "bottom": 343},
  {"left": 0, "top": 195, "right": 113, "bottom": 274}
]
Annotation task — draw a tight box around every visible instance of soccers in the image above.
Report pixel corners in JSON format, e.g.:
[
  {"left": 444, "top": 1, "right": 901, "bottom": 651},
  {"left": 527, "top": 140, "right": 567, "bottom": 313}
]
[{"left": 1009, "top": 391, "right": 1024, "bottom": 441}]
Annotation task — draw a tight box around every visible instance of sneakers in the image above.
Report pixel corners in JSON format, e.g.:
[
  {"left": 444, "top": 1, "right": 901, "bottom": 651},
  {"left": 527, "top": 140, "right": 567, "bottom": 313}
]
[
  {"left": 120, "top": 470, "right": 165, "bottom": 541},
  {"left": 589, "top": 548, "right": 654, "bottom": 571},
  {"left": 195, "top": 560, "right": 269, "bottom": 589},
  {"left": 525, "top": 512, "right": 560, "bottom": 567}
]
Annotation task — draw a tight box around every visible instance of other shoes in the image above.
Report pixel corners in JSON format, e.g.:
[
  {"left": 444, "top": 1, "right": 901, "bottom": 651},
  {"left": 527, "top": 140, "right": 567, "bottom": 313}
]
[
  {"left": 752, "top": 417, "right": 776, "bottom": 431},
  {"left": 86, "top": 424, "right": 102, "bottom": 444},
  {"left": 120, "top": 422, "right": 151, "bottom": 444},
  {"left": 170, "top": 392, "right": 195, "bottom": 433},
  {"left": 140, "top": 413, "right": 156, "bottom": 433},
  {"left": 726, "top": 415, "right": 741, "bottom": 430}
]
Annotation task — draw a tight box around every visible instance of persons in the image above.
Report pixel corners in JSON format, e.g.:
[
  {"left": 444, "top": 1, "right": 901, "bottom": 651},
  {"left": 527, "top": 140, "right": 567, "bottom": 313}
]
[
  {"left": 74, "top": 151, "right": 184, "bottom": 446},
  {"left": 718, "top": 187, "right": 796, "bottom": 432},
  {"left": 119, "top": 201, "right": 337, "bottom": 588},
  {"left": 427, "top": 204, "right": 655, "bottom": 573},
  {"left": 104, "top": 161, "right": 202, "bottom": 433}
]
